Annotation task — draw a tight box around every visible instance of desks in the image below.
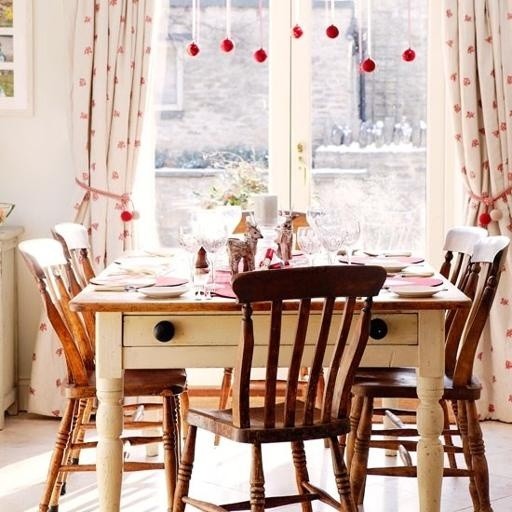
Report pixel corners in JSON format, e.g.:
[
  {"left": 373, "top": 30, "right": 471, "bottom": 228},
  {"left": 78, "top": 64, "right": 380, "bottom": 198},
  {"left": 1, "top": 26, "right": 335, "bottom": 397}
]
[{"left": 68, "top": 245, "right": 473, "bottom": 512}]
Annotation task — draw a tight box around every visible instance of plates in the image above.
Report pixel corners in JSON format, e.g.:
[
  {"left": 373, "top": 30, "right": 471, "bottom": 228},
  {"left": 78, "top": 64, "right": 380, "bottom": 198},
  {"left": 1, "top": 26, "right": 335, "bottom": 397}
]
[
  {"left": 338, "top": 245, "right": 441, "bottom": 299},
  {"left": 89, "top": 255, "right": 188, "bottom": 302}
]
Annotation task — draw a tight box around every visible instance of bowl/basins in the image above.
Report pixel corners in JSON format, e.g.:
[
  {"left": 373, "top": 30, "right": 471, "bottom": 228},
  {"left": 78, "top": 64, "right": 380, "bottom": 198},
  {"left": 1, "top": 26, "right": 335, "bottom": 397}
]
[{"left": 0, "top": 202, "right": 17, "bottom": 225}]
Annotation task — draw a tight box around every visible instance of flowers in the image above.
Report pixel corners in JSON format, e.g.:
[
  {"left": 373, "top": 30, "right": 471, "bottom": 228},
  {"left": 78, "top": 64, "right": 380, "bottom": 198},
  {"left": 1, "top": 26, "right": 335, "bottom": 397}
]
[{"left": 194, "top": 161, "right": 267, "bottom": 212}]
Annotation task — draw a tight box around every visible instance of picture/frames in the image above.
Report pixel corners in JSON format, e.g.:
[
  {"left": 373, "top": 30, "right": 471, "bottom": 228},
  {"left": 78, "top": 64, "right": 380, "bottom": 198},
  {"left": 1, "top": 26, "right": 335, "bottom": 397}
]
[{"left": 0, "top": 0, "right": 34, "bottom": 111}]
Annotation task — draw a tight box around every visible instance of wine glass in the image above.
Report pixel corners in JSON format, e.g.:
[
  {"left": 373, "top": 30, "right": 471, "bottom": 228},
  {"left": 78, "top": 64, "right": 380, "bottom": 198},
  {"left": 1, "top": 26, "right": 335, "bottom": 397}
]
[{"left": 296, "top": 194, "right": 358, "bottom": 265}]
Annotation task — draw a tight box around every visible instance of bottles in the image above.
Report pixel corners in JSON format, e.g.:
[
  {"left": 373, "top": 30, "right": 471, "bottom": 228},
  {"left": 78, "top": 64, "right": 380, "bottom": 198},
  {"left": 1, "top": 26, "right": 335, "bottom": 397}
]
[
  {"left": 0, "top": 44, "right": 6, "bottom": 63},
  {"left": 259, "top": 247, "right": 284, "bottom": 270},
  {"left": 0, "top": 70, "right": 14, "bottom": 97},
  {"left": 193, "top": 247, "right": 211, "bottom": 300}
]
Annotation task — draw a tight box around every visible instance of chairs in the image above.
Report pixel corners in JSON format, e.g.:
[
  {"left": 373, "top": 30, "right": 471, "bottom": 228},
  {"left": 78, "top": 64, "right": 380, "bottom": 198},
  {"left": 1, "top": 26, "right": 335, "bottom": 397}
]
[
  {"left": 52, "top": 222, "right": 187, "bottom": 504},
  {"left": 344, "top": 226, "right": 491, "bottom": 476},
  {"left": 171, "top": 266, "right": 389, "bottom": 512},
  {"left": 15, "top": 238, "right": 189, "bottom": 512},
  {"left": 342, "top": 237, "right": 512, "bottom": 512}
]
